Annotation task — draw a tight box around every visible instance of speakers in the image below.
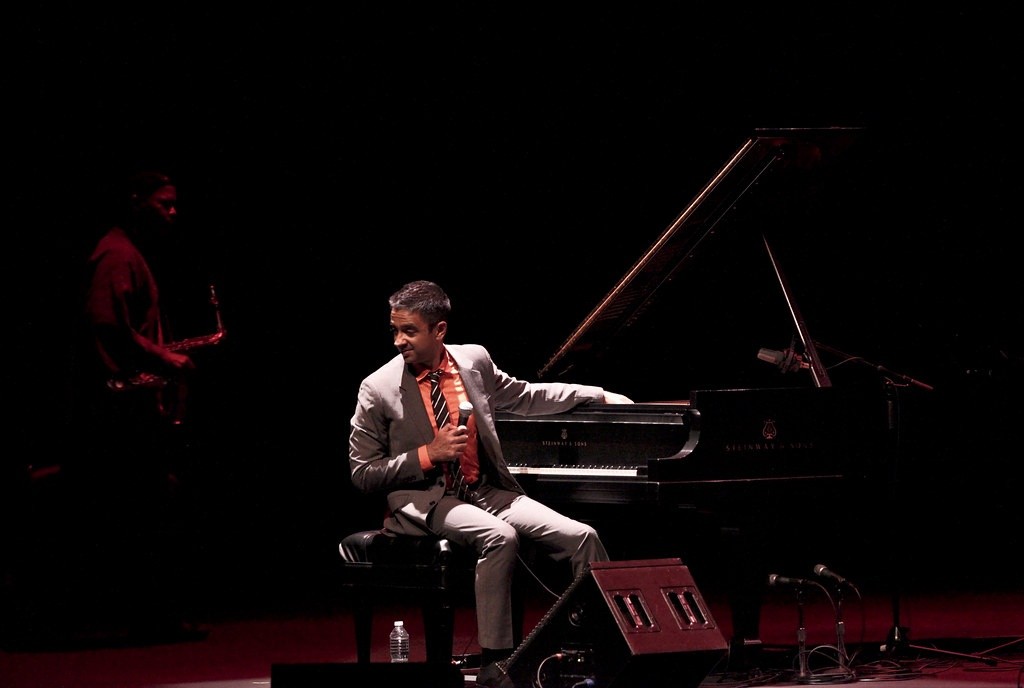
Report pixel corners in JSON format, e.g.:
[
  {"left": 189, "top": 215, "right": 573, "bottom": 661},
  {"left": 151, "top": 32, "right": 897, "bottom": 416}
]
[{"left": 503, "top": 557, "right": 728, "bottom": 688}]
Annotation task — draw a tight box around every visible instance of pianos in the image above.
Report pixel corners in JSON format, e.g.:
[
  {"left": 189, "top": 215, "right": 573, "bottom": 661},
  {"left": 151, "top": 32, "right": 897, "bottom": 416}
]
[{"left": 495, "top": 124, "right": 920, "bottom": 671}]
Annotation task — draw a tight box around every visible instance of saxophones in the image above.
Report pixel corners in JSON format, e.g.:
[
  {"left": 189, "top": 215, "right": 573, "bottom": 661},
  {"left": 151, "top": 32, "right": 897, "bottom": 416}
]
[{"left": 164, "top": 283, "right": 229, "bottom": 352}]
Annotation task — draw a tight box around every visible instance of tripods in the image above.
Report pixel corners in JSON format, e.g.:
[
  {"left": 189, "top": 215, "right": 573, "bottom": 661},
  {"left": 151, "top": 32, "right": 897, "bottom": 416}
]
[{"left": 790, "top": 335, "right": 998, "bottom": 666}]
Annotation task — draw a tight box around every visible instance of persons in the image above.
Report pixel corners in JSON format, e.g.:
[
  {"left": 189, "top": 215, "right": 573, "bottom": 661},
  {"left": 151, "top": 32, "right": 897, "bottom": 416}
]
[
  {"left": 349, "top": 280, "right": 634, "bottom": 688},
  {"left": 80, "top": 167, "right": 196, "bottom": 637}
]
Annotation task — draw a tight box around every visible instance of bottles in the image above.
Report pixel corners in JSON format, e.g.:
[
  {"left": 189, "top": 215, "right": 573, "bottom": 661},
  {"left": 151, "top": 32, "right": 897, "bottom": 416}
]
[{"left": 390, "top": 621, "right": 409, "bottom": 663}]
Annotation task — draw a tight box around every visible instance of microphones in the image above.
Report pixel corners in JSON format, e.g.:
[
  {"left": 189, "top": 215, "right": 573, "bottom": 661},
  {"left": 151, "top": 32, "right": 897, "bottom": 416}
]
[
  {"left": 757, "top": 348, "right": 810, "bottom": 370},
  {"left": 814, "top": 564, "right": 856, "bottom": 590},
  {"left": 768, "top": 574, "right": 818, "bottom": 586},
  {"left": 448, "top": 401, "right": 474, "bottom": 470}
]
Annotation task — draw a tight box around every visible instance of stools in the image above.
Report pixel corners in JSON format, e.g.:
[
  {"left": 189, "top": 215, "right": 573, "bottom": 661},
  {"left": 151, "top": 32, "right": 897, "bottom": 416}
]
[{"left": 339, "top": 529, "right": 520, "bottom": 663}]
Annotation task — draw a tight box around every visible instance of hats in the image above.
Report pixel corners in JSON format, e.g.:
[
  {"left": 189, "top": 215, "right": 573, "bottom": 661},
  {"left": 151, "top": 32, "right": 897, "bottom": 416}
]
[{"left": 141, "top": 170, "right": 178, "bottom": 195}]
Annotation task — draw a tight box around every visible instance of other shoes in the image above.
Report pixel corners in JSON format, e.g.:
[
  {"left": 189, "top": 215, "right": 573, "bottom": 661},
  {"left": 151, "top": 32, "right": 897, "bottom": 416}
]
[
  {"left": 92, "top": 611, "right": 209, "bottom": 648},
  {"left": 476, "top": 661, "right": 515, "bottom": 688}
]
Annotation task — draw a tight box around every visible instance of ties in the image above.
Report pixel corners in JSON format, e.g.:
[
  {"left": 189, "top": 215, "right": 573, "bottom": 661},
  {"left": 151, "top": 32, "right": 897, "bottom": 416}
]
[{"left": 427, "top": 370, "right": 472, "bottom": 502}]
